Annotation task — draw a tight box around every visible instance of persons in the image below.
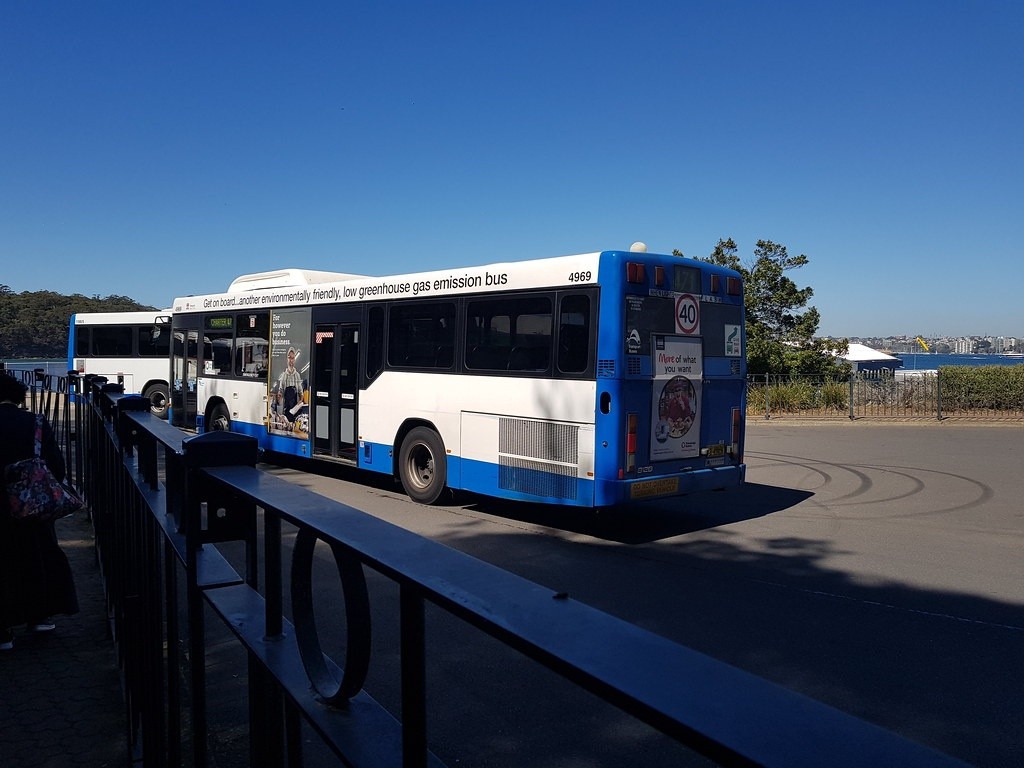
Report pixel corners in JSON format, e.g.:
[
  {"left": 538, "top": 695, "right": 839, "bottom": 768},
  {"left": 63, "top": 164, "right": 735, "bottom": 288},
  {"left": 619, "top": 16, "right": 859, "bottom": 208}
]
[
  {"left": 0, "top": 371, "right": 68, "bottom": 652},
  {"left": 270, "top": 346, "right": 303, "bottom": 421}
]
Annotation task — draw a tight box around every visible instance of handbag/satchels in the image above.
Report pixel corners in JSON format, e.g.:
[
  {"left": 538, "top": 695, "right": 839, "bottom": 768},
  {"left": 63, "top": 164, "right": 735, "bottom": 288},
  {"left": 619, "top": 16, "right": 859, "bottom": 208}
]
[{"left": 4, "top": 414, "right": 83, "bottom": 522}]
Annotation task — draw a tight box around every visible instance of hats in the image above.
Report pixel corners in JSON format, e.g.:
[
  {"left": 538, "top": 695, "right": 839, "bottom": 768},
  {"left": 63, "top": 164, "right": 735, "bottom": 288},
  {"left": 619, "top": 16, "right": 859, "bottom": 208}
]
[{"left": 287, "top": 347, "right": 296, "bottom": 356}]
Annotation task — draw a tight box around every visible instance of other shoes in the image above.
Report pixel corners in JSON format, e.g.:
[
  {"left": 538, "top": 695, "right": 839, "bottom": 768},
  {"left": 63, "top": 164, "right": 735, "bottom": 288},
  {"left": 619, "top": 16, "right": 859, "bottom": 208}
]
[
  {"left": 67, "top": 604, "right": 79, "bottom": 614},
  {"left": 26, "top": 618, "right": 56, "bottom": 632},
  {"left": 0, "top": 631, "right": 15, "bottom": 649}
]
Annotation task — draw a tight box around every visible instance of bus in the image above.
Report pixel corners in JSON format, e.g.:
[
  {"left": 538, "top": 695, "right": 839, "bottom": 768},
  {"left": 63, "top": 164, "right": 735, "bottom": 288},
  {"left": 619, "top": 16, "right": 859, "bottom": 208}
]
[
  {"left": 210, "top": 336, "right": 269, "bottom": 378},
  {"left": 150, "top": 242, "right": 748, "bottom": 509},
  {"left": 174, "top": 332, "right": 212, "bottom": 394},
  {"left": 67, "top": 308, "right": 175, "bottom": 418}
]
